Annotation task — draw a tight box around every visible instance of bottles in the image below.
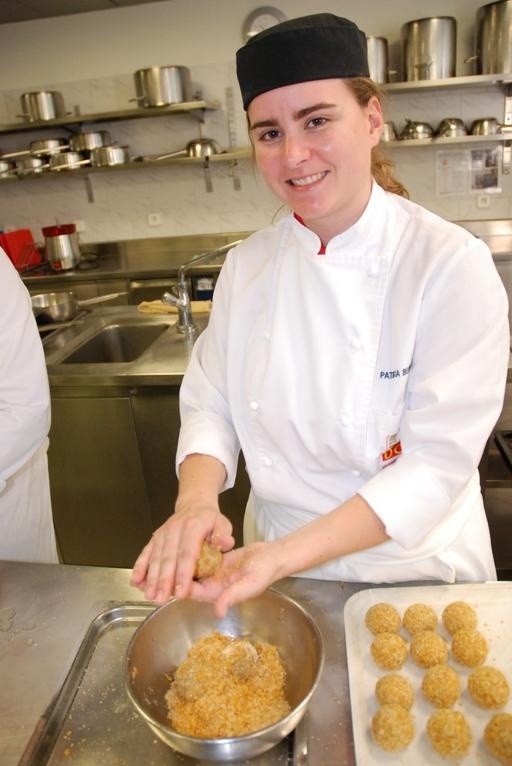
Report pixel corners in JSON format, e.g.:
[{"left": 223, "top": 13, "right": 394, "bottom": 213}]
[{"left": 42, "top": 223, "right": 82, "bottom": 272}]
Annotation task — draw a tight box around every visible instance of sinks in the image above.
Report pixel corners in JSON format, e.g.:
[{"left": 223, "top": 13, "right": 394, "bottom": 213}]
[{"left": 44, "top": 317, "right": 177, "bottom": 367}]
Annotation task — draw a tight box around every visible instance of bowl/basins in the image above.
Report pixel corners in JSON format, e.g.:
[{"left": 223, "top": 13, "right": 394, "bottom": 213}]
[{"left": 123, "top": 583, "right": 326, "bottom": 764}]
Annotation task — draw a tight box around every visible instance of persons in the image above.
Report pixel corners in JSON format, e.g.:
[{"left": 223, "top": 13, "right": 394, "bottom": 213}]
[
  {"left": 127, "top": 14, "right": 510, "bottom": 617},
  {"left": 0, "top": 247, "right": 64, "bottom": 566}
]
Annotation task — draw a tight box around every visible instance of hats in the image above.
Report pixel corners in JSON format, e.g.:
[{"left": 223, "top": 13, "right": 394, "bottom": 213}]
[{"left": 237, "top": 13, "right": 370, "bottom": 111}]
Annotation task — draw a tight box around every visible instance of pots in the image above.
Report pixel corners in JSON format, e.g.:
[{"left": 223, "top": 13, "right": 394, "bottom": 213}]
[
  {"left": 399, "top": 14, "right": 456, "bottom": 80},
  {"left": 31, "top": 290, "right": 130, "bottom": 322},
  {"left": 382, "top": 113, "right": 512, "bottom": 142},
  {"left": 129, "top": 65, "right": 199, "bottom": 111},
  {"left": 366, "top": 33, "right": 398, "bottom": 82},
  {"left": 156, "top": 137, "right": 225, "bottom": 162},
  {"left": 15, "top": 85, "right": 72, "bottom": 120},
  {"left": 1, "top": 130, "right": 129, "bottom": 177},
  {"left": 465, "top": 0, "right": 511, "bottom": 71}
]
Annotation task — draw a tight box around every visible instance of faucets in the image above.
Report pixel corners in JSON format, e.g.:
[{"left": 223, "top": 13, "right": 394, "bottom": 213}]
[{"left": 162, "top": 237, "right": 246, "bottom": 332}]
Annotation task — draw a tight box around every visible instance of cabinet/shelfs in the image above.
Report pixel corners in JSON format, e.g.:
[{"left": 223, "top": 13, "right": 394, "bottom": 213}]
[
  {"left": 214, "top": 131, "right": 511, "bottom": 181},
  {"left": 1, "top": 100, "right": 254, "bottom": 183},
  {"left": 379, "top": 72, "right": 512, "bottom": 92}
]
